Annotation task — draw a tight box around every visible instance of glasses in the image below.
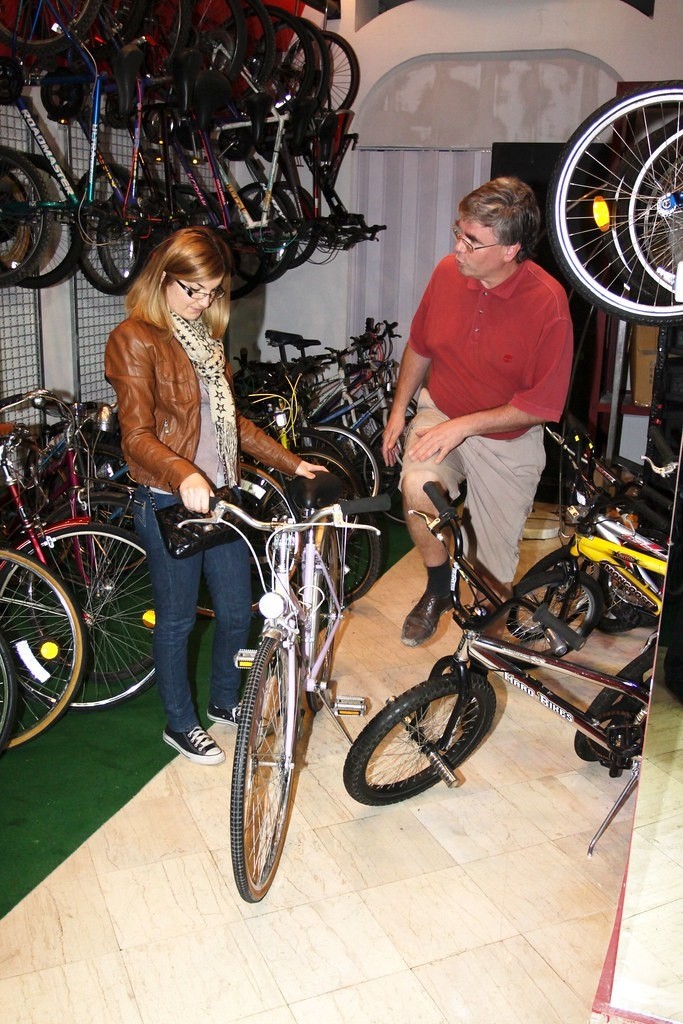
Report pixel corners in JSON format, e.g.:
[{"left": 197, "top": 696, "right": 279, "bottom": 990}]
[
  {"left": 175, "top": 278, "right": 225, "bottom": 299},
  {"left": 451, "top": 224, "right": 499, "bottom": 254}
]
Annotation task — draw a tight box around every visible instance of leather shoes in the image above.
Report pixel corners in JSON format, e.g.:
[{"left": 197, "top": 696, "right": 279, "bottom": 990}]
[
  {"left": 460, "top": 696, "right": 481, "bottom": 733},
  {"left": 400, "top": 585, "right": 455, "bottom": 647}
]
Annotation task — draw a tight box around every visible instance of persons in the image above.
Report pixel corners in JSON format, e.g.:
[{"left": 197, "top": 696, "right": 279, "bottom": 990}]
[
  {"left": 104, "top": 226, "right": 329, "bottom": 766},
  {"left": 382, "top": 177, "right": 574, "bottom": 732}
]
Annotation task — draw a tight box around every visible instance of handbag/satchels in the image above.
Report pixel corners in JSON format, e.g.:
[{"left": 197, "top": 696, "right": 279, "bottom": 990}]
[{"left": 154, "top": 485, "right": 246, "bottom": 560}]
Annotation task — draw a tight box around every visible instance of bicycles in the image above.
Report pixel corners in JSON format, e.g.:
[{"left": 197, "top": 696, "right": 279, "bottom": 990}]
[{"left": 0, "top": 1, "right": 683, "bottom": 904}]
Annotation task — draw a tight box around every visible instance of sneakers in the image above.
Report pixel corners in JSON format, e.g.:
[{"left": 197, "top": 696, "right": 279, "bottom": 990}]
[
  {"left": 162, "top": 724, "right": 226, "bottom": 765},
  {"left": 207, "top": 699, "right": 275, "bottom": 736}
]
